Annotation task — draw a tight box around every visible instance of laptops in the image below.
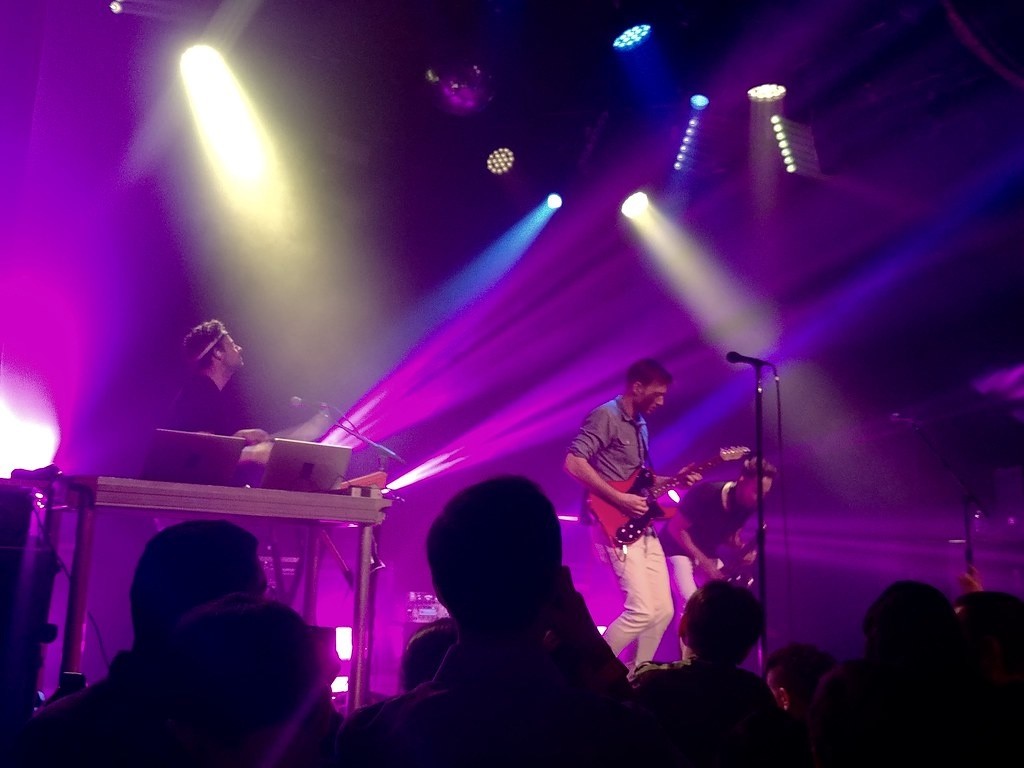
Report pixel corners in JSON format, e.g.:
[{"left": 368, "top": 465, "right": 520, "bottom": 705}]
[
  {"left": 260, "top": 438, "right": 352, "bottom": 494},
  {"left": 137, "top": 428, "right": 246, "bottom": 486}
]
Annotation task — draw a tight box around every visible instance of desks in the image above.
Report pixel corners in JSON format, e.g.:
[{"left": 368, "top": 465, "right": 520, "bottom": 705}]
[{"left": 38, "top": 470, "right": 393, "bottom": 721}]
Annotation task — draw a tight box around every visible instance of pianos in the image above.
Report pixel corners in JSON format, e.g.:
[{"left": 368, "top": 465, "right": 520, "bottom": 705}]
[{"left": 10, "top": 437, "right": 391, "bottom": 728}]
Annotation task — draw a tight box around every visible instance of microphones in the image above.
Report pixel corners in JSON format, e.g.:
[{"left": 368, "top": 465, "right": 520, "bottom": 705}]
[
  {"left": 291, "top": 396, "right": 331, "bottom": 410},
  {"left": 726, "top": 351, "right": 770, "bottom": 367},
  {"left": 889, "top": 413, "right": 919, "bottom": 427}
]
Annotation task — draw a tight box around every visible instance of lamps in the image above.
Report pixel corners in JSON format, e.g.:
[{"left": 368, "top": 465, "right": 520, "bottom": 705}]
[{"left": 484, "top": 8, "right": 787, "bottom": 176}]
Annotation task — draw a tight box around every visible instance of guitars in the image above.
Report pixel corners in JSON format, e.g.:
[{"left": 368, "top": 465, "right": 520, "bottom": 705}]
[{"left": 583, "top": 444, "right": 751, "bottom": 549}]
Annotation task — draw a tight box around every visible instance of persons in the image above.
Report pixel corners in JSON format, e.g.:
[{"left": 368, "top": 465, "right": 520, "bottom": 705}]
[
  {"left": 562, "top": 358, "right": 703, "bottom": 675},
  {"left": 0, "top": 474, "right": 1024, "bottom": 768},
  {"left": 142, "top": 320, "right": 273, "bottom": 531},
  {"left": 659, "top": 457, "right": 778, "bottom": 662}
]
